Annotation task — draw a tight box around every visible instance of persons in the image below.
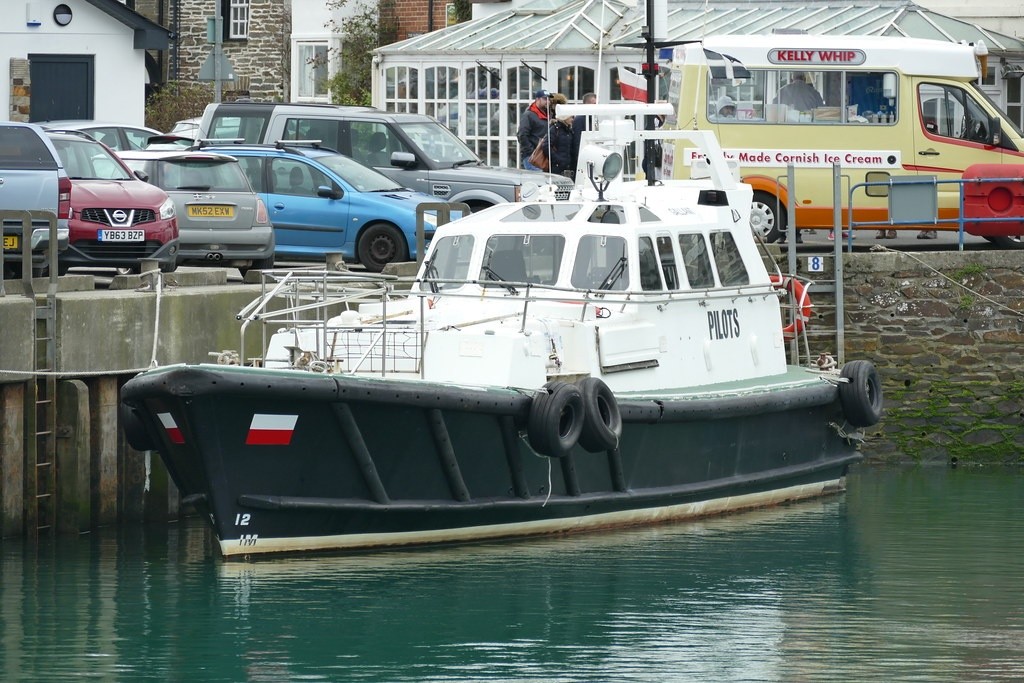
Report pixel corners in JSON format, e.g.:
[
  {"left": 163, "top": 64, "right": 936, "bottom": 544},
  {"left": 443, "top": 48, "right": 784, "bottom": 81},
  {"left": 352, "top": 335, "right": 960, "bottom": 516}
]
[
  {"left": 440, "top": 77, "right": 527, "bottom": 163},
  {"left": 993, "top": 133, "right": 1000, "bottom": 145},
  {"left": 708, "top": 96, "right": 735, "bottom": 120},
  {"left": 518, "top": 89, "right": 665, "bottom": 184},
  {"left": 774, "top": 71, "right": 825, "bottom": 113},
  {"left": 773, "top": 229, "right": 940, "bottom": 242}
]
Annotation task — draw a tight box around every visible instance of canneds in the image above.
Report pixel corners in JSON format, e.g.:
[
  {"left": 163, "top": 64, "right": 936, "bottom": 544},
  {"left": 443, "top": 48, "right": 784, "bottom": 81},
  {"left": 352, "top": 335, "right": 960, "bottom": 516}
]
[{"left": 871, "top": 113, "right": 894, "bottom": 124}]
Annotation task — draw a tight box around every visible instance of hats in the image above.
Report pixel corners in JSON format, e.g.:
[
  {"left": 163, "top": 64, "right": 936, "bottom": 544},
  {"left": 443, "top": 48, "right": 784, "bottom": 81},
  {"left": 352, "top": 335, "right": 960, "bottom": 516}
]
[
  {"left": 716, "top": 96, "right": 735, "bottom": 113},
  {"left": 554, "top": 104, "right": 574, "bottom": 120},
  {"left": 535, "top": 89, "right": 554, "bottom": 98}
]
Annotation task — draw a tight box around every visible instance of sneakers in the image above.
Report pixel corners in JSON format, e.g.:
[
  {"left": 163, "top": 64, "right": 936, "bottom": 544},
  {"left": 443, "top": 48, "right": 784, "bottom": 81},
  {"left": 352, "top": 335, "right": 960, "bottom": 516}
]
[
  {"left": 827, "top": 232, "right": 834, "bottom": 240},
  {"left": 842, "top": 232, "right": 856, "bottom": 238}
]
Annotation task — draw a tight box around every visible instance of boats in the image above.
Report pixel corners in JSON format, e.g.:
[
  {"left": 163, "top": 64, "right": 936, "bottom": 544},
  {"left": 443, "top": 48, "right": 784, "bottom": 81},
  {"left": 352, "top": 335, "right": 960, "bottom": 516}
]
[{"left": 120, "top": 0, "right": 884, "bottom": 565}]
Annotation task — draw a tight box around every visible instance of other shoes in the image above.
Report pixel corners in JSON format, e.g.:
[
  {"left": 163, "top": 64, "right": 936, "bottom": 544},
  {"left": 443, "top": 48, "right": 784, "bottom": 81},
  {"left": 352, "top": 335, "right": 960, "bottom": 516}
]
[
  {"left": 924, "top": 231, "right": 937, "bottom": 239},
  {"left": 875, "top": 230, "right": 885, "bottom": 238},
  {"left": 886, "top": 230, "right": 897, "bottom": 238},
  {"left": 776, "top": 236, "right": 786, "bottom": 243},
  {"left": 796, "top": 234, "right": 803, "bottom": 243},
  {"left": 809, "top": 230, "right": 816, "bottom": 234},
  {"left": 916, "top": 230, "right": 928, "bottom": 239}
]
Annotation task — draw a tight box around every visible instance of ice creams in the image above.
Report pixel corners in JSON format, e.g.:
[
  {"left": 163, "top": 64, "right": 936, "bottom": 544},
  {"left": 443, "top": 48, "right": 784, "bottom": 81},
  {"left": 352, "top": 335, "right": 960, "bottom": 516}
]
[{"left": 976, "top": 39, "right": 989, "bottom": 78}]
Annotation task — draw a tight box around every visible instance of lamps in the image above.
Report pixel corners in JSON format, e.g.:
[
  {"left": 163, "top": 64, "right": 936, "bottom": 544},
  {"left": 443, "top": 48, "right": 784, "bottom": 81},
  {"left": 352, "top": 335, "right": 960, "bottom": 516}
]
[
  {"left": 54, "top": 4, "right": 72, "bottom": 26},
  {"left": 999, "top": 57, "right": 1024, "bottom": 80}
]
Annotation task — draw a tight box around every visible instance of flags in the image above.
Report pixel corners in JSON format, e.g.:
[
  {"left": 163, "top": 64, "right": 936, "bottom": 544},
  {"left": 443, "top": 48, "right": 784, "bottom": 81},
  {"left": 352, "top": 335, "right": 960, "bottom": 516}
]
[{"left": 616, "top": 61, "right": 649, "bottom": 103}]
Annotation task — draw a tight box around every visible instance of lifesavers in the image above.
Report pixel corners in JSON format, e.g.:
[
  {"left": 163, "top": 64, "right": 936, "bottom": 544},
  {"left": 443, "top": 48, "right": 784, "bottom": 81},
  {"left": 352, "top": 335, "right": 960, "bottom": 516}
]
[
  {"left": 571, "top": 376, "right": 624, "bottom": 454},
  {"left": 769, "top": 276, "right": 813, "bottom": 342},
  {"left": 527, "top": 380, "right": 585, "bottom": 458},
  {"left": 839, "top": 360, "right": 884, "bottom": 427},
  {"left": 120, "top": 402, "right": 154, "bottom": 452}
]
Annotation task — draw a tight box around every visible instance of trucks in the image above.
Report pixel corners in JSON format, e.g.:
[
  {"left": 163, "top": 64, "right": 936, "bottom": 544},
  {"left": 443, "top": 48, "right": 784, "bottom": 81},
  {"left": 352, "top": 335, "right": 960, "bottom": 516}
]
[{"left": 662, "top": 34, "right": 1024, "bottom": 246}]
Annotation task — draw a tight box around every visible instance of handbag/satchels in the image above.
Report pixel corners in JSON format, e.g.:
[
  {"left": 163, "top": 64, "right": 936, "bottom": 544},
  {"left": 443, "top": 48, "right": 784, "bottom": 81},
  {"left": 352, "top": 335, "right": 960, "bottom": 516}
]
[
  {"left": 653, "top": 139, "right": 663, "bottom": 167},
  {"left": 528, "top": 123, "right": 559, "bottom": 171}
]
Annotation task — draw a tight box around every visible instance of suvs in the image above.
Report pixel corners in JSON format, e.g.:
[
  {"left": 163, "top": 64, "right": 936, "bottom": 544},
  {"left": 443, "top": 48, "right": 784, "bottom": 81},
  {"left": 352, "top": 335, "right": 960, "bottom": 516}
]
[{"left": 1, "top": 102, "right": 573, "bottom": 278}]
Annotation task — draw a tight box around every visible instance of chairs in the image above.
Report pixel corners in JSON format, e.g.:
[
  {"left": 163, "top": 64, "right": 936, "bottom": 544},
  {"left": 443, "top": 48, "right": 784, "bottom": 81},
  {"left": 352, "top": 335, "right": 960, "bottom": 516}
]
[
  {"left": 181, "top": 171, "right": 205, "bottom": 185},
  {"left": 350, "top": 129, "right": 363, "bottom": 160},
  {"left": 367, "top": 132, "right": 388, "bottom": 165},
  {"left": 491, "top": 250, "right": 528, "bottom": 283},
  {"left": 290, "top": 167, "right": 303, "bottom": 192},
  {"left": 101, "top": 133, "right": 118, "bottom": 151}
]
